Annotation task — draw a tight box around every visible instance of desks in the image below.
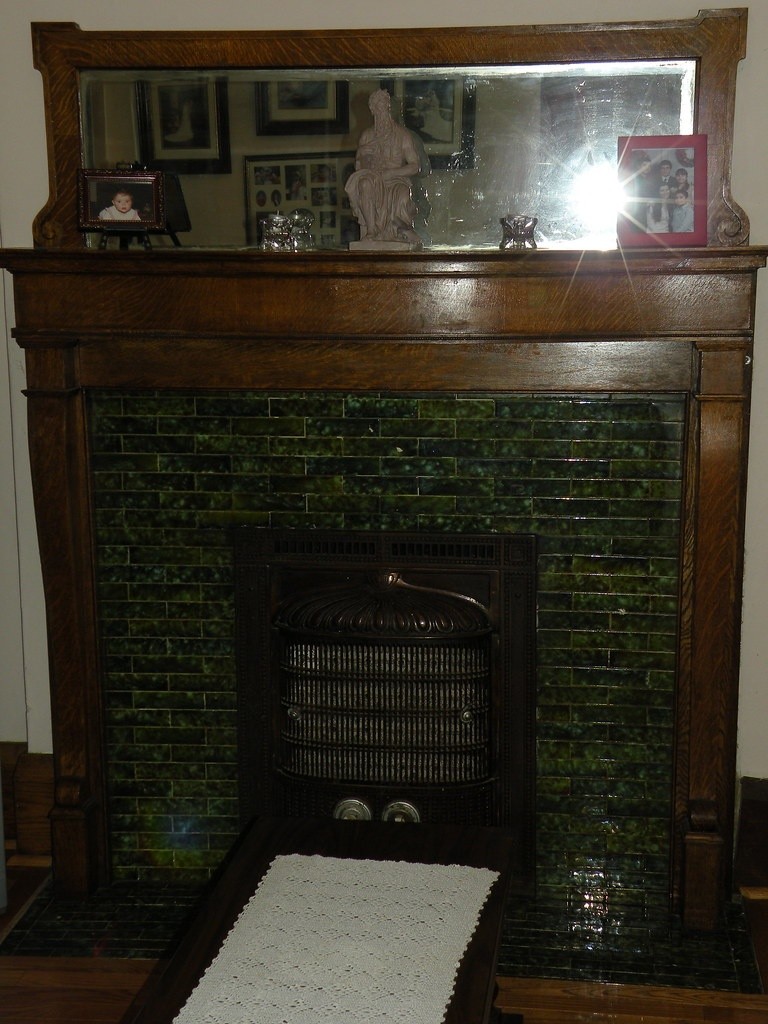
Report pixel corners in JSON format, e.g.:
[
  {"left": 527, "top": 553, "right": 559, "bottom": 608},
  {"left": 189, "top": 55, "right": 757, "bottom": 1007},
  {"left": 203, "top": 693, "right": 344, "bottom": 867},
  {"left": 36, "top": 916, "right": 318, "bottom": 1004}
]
[{"left": 119, "top": 814, "right": 529, "bottom": 1024}]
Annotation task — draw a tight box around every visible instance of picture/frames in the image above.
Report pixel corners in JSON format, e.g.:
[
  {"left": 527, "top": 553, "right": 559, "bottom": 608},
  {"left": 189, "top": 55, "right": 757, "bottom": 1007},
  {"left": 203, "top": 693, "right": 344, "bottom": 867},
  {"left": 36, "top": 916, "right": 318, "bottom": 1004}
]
[
  {"left": 616, "top": 134, "right": 707, "bottom": 248},
  {"left": 380, "top": 79, "right": 476, "bottom": 169},
  {"left": 135, "top": 77, "right": 232, "bottom": 174},
  {"left": 255, "top": 80, "right": 349, "bottom": 135},
  {"left": 242, "top": 151, "right": 361, "bottom": 246},
  {"left": 78, "top": 168, "right": 165, "bottom": 235}
]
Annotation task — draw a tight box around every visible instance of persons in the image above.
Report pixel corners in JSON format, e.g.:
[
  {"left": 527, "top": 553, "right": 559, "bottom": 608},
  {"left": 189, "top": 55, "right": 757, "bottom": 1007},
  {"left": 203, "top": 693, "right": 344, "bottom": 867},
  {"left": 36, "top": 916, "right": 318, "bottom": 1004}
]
[
  {"left": 404, "top": 126, "right": 433, "bottom": 246},
  {"left": 98, "top": 187, "right": 141, "bottom": 221},
  {"left": 627, "top": 150, "right": 695, "bottom": 233},
  {"left": 343, "top": 88, "right": 423, "bottom": 244},
  {"left": 142, "top": 78, "right": 454, "bottom": 247}
]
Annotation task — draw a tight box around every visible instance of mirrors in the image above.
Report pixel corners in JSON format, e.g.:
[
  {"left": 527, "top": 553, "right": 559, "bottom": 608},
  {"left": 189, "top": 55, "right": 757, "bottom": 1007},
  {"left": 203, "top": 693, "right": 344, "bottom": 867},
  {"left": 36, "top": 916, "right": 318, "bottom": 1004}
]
[{"left": 30, "top": 6, "right": 748, "bottom": 251}]
